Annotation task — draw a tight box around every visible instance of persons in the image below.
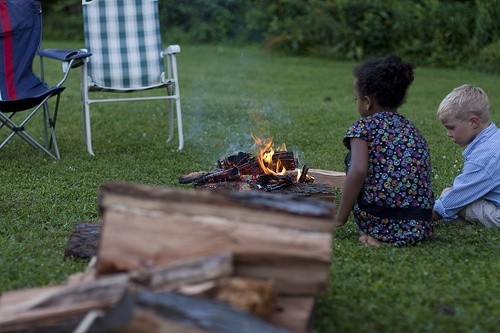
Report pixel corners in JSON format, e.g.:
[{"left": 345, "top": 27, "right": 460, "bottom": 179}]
[
  {"left": 335, "top": 54, "right": 436, "bottom": 249},
  {"left": 432, "top": 83, "right": 500, "bottom": 227}
]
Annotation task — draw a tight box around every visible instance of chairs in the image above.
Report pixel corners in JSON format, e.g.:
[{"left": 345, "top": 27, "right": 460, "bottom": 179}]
[
  {"left": 0, "top": 0, "right": 93, "bottom": 160},
  {"left": 81, "top": 0, "right": 184, "bottom": 157}
]
